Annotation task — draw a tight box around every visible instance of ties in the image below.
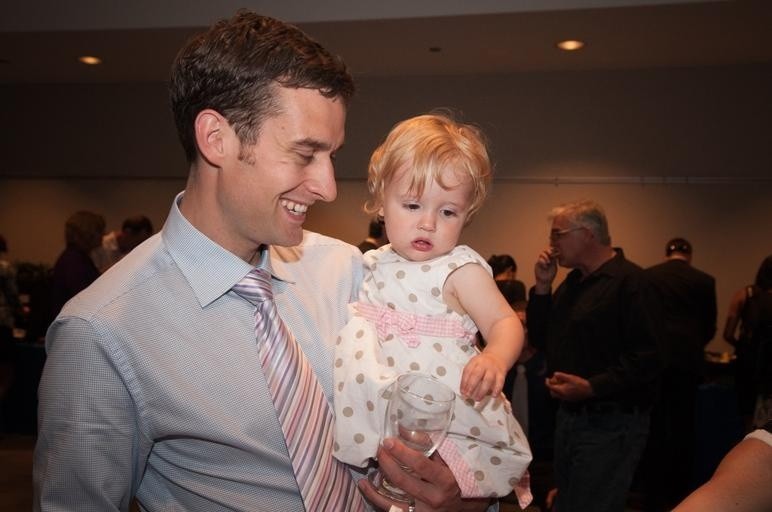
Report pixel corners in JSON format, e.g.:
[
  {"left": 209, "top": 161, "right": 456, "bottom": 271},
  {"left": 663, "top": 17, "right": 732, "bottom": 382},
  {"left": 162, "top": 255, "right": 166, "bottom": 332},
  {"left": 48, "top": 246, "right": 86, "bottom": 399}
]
[{"left": 230, "top": 268, "right": 370, "bottom": 512}]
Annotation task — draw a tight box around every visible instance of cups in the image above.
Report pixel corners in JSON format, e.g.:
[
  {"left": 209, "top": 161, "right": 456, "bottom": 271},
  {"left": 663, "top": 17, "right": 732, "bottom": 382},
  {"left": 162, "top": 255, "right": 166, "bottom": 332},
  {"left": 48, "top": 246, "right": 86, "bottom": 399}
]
[{"left": 368, "top": 372, "right": 457, "bottom": 503}]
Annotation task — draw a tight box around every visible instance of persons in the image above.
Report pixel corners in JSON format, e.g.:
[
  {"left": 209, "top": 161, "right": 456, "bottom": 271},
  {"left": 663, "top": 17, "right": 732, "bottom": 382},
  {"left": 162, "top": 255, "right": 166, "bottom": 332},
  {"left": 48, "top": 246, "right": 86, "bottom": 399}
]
[
  {"left": 1, "top": 235, "right": 31, "bottom": 397},
  {"left": 523, "top": 196, "right": 665, "bottom": 511},
  {"left": 322, "top": 103, "right": 539, "bottom": 510},
  {"left": 29, "top": 5, "right": 513, "bottom": 512},
  {"left": 47, "top": 210, "right": 105, "bottom": 335},
  {"left": 474, "top": 253, "right": 526, "bottom": 403},
  {"left": 670, "top": 417, "right": 771, "bottom": 511},
  {"left": 721, "top": 255, "right": 772, "bottom": 427},
  {"left": 88, "top": 214, "right": 155, "bottom": 276},
  {"left": 356, "top": 216, "right": 391, "bottom": 254},
  {"left": 643, "top": 237, "right": 718, "bottom": 442}
]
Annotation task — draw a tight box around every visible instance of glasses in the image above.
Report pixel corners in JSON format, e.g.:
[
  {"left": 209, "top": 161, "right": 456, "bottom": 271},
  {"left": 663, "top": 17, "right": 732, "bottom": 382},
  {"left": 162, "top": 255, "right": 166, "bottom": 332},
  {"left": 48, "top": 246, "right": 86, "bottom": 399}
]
[{"left": 548, "top": 224, "right": 590, "bottom": 241}]
